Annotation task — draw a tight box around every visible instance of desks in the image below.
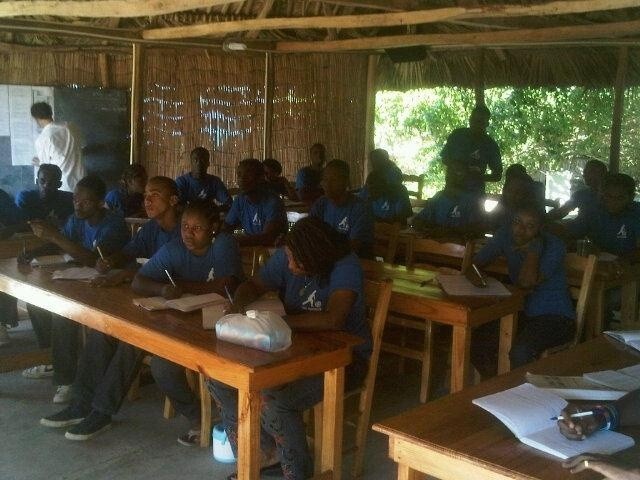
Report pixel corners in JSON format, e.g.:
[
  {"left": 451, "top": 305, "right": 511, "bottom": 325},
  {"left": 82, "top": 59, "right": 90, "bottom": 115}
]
[{"left": 0, "top": 195, "right": 638, "bottom": 480}]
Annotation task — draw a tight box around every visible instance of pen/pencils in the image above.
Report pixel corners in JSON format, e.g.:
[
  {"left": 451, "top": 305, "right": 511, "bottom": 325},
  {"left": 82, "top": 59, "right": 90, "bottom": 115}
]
[
  {"left": 472, "top": 264, "right": 487, "bottom": 288},
  {"left": 165, "top": 270, "right": 176, "bottom": 286},
  {"left": 96, "top": 246, "right": 109, "bottom": 269},
  {"left": 225, "top": 286, "right": 233, "bottom": 305},
  {"left": 550, "top": 410, "right": 602, "bottom": 420}
]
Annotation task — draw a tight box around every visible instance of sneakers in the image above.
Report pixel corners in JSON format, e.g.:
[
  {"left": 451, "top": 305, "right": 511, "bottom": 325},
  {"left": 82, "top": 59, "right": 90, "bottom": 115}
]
[
  {"left": 22, "top": 363, "right": 54, "bottom": 378},
  {"left": 40, "top": 403, "right": 94, "bottom": 428},
  {"left": 65, "top": 410, "right": 112, "bottom": 441},
  {"left": 0, "top": 320, "right": 12, "bottom": 347},
  {"left": 53, "top": 384, "right": 74, "bottom": 403},
  {"left": 177, "top": 422, "right": 210, "bottom": 446}
]
